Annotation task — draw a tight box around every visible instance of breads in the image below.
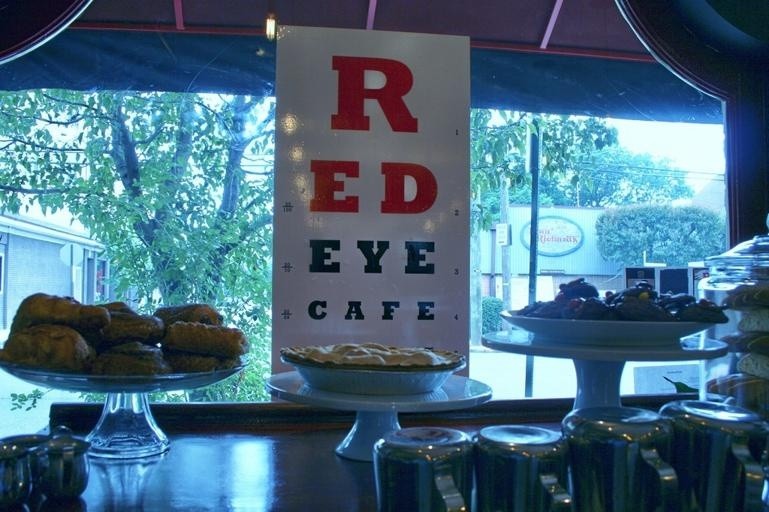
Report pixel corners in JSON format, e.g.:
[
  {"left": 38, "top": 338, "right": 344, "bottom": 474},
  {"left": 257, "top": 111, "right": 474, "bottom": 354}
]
[
  {"left": 706, "top": 290, "right": 769, "bottom": 421},
  {"left": 0, "top": 291, "right": 249, "bottom": 373},
  {"left": 519, "top": 276, "right": 729, "bottom": 324}
]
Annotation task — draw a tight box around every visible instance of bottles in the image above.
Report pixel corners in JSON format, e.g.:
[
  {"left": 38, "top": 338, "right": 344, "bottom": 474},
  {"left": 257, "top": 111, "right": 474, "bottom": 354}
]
[{"left": 697, "top": 234, "right": 769, "bottom": 400}]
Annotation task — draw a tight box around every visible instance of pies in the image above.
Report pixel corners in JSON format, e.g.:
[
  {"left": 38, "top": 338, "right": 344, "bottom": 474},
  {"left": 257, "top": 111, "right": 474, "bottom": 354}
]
[{"left": 280, "top": 342, "right": 464, "bottom": 371}]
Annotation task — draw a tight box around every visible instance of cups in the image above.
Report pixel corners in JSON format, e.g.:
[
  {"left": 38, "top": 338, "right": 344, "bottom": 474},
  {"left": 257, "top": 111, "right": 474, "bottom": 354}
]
[
  {"left": 560, "top": 406, "right": 679, "bottom": 511},
  {"left": 470, "top": 423, "right": 572, "bottom": 511},
  {"left": 372, "top": 426, "right": 474, "bottom": 511},
  {"left": 333, "top": 401, "right": 402, "bottom": 462},
  {"left": 1, "top": 424, "right": 92, "bottom": 510},
  {"left": 660, "top": 399, "right": 764, "bottom": 512},
  {"left": 499, "top": 309, "right": 728, "bottom": 348}
]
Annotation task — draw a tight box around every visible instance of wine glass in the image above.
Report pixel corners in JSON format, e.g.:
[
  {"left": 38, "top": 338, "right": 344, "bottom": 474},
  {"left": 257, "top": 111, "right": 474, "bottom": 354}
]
[{"left": 0, "top": 363, "right": 251, "bottom": 460}]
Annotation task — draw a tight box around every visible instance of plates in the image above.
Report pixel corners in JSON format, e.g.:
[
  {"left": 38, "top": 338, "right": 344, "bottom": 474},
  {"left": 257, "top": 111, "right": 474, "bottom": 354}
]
[{"left": 278, "top": 355, "right": 468, "bottom": 395}]
